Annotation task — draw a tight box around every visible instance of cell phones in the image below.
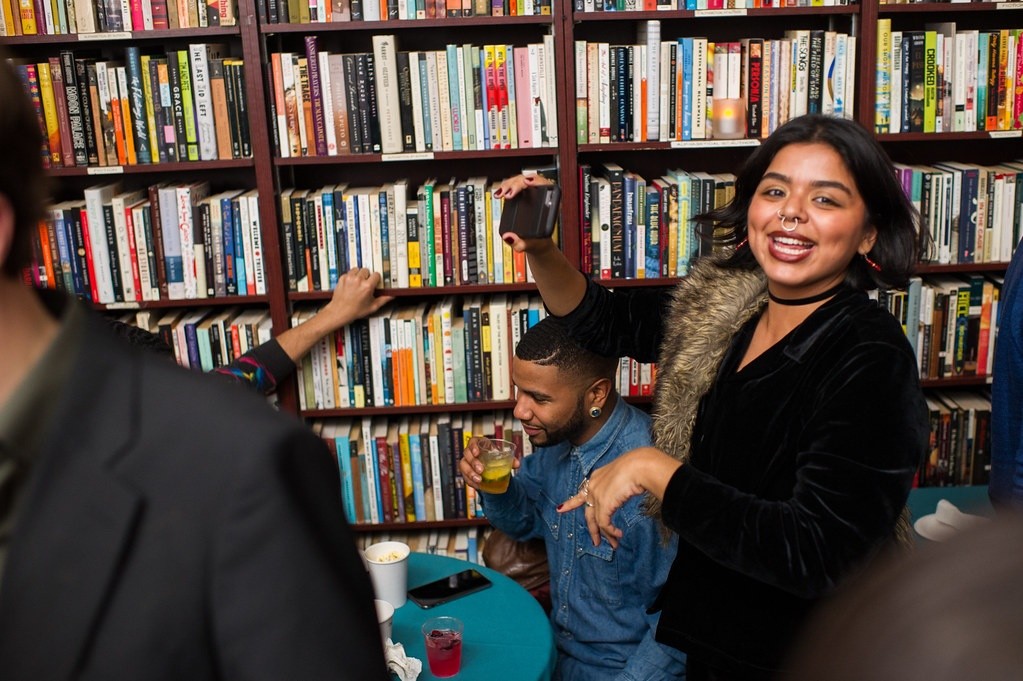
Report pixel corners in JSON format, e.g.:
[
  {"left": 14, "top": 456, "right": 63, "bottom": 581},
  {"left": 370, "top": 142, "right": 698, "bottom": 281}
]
[
  {"left": 500, "top": 184, "right": 560, "bottom": 239},
  {"left": 407, "top": 569, "right": 492, "bottom": 609}
]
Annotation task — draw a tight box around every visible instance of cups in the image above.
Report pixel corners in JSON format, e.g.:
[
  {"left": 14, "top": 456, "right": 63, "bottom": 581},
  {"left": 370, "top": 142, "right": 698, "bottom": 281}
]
[
  {"left": 477, "top": 439, "right": 516, "bottom": 494},
  {"left": 711, "top": 97, "right": 747, "bottom": 138},
  {"left": 373, "top": 599, "right": 394, "bottom": 639},
  {"left": 421, "top": 616, "right": 463, "bottom": 677},
  {"left": 365, "top": 542, "right": 411, "bottom": 609}
]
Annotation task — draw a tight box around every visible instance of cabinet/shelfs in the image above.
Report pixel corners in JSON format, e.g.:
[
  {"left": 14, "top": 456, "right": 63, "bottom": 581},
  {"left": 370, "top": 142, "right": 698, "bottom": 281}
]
[{"left": 0, "top": 0, "right": 1023, "bottom": 542}]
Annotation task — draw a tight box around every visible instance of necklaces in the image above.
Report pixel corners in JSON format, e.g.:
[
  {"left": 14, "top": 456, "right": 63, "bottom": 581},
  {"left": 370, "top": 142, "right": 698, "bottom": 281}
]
[{"left": 766, "top": 278, "right": 845, "bottom": 306}]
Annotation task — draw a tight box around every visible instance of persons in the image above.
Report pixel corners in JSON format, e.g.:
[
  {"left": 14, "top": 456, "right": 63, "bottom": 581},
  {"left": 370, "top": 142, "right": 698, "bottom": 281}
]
[
  {"left": 208, "top": 266, "right": 393, "bottom": 395},
  {"left": 493, "top": 112, "right": 936, "bottom": 681},
  {"left": 990, "top": 236, "right": 1023, "bottom": 514},
  {"left": 0, "top": 58, "right": 393, "bottom": 681},
  {"left": 458, "top": 316, "right": 687, "bottom": 681}
]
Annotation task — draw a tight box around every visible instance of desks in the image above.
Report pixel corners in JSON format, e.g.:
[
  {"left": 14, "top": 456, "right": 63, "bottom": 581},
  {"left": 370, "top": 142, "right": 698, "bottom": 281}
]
[
  {"left": 904, "top": 485, "right": 996, "bottom": 550},
  {"left": 391, "top": 552, "right": 557, "bottom": 681}
]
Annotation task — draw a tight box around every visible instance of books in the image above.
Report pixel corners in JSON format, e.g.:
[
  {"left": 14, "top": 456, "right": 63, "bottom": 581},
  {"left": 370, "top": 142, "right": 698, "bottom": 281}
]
[
  {"left": 876, "top": 0, "right": 1023, "bottom": 487},
  {"left": 0, "top": 0, "right": 271, "bottom": 371},
  {"left": 291, "top": 297, "right": 553, "bottom": 575},
  {"left": 614, "top": 356, "right": 661, "bottom": 399},
  {"left": 259, "top": 0, "right": 556, "bottom": 291},
  {"left": 576, "top": 0, "right": 858, "bottom": 277}
]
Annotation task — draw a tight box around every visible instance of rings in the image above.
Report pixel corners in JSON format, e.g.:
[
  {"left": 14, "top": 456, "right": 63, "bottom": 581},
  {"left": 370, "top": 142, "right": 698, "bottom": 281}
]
[
  {"left": 585, "top": 501, "right": 595, "bottom": 508},
  {"left": 578, "top": 477, "right": 591, "bottom": 497}
]
